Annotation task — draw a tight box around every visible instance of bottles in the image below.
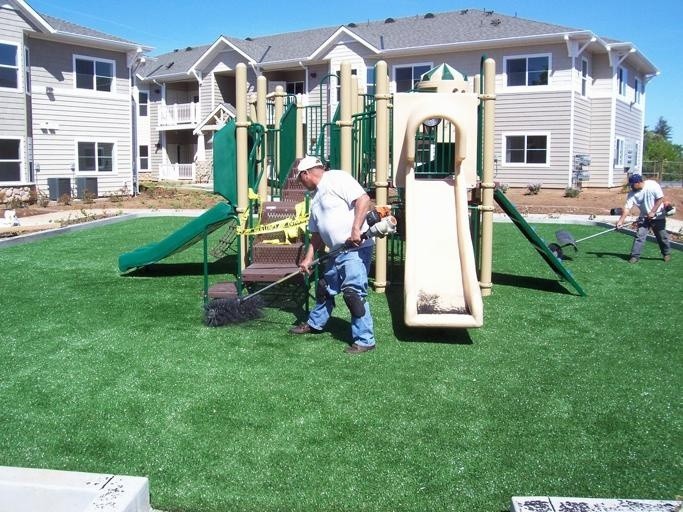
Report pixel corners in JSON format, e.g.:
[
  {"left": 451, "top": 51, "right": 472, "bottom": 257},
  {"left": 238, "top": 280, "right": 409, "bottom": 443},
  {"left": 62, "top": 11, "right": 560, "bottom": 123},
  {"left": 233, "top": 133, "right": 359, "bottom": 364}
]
[
  {"left": 294, "top": 155, "right": 324, "bottom": 185},
  {"left": 624, "top": 174, "right": 643, "bottom": 187}
]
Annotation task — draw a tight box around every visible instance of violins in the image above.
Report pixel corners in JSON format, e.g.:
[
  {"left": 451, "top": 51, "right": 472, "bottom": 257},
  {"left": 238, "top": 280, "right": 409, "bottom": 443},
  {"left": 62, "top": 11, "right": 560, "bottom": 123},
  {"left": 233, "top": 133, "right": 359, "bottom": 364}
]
[
  {"left": 118, "top": 202, "right": 237, "bottom": 272},
  {"left": 405, "top": 167, "right": 483, "bottom": 328}
]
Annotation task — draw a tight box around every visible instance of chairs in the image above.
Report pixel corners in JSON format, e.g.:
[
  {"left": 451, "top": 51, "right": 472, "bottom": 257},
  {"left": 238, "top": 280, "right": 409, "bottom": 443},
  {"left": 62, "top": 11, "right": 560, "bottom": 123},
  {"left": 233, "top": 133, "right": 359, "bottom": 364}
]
[
  {"left": 628, "top": 256, "right": 639, "bottom": 264},
  {"left": 662, "top": 255, "right": 671, "bottom": 262},
  {"left": 343, "top": 340, "right": 376, "bottom": 355},
  {"left": 288, "top": 324, "right": 325, "bottom": 335}
]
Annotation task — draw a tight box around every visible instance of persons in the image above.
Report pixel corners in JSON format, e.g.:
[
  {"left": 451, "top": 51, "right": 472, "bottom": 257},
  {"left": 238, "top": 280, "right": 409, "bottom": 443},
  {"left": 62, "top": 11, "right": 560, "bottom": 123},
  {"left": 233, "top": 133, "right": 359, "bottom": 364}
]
[
  {"left": 290, "top": 156, "right": 376, "bottom": 354},
  {"left": 616, "top": 174, "right": 671, "bottom": 264}
]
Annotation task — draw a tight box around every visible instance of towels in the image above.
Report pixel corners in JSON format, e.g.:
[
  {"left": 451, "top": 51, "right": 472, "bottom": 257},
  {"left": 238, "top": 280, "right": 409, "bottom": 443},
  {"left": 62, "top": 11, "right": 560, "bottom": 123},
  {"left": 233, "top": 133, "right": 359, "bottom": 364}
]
[
  {"left": 47, "top": 178, "right": 71, "bottom": 201},
  {"left": 75, "top": 177, "right": 98, "bottom": 199}
]
